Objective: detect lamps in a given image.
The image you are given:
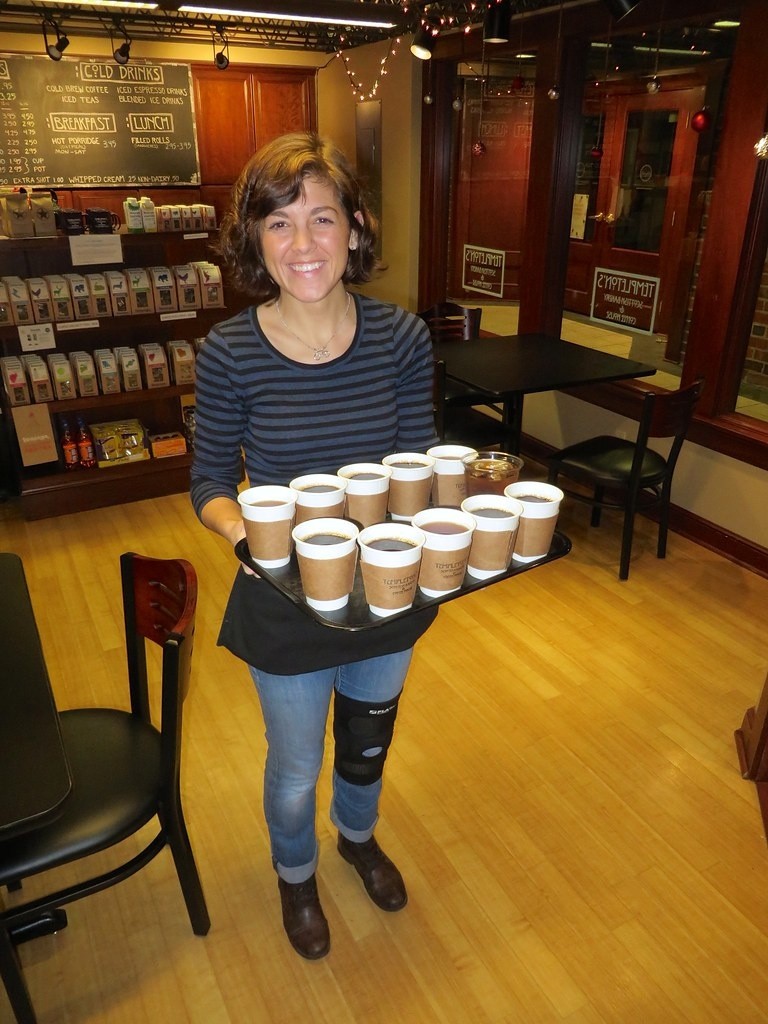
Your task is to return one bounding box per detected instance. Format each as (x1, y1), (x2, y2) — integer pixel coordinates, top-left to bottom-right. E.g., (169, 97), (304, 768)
(107, 15), (132, 65)
(482, 0), (512, 43)
(608, 0), (639, 20)
(410, 0), (444, 61)
(41, 11), (68, 60)
(213, 21), (232, 70)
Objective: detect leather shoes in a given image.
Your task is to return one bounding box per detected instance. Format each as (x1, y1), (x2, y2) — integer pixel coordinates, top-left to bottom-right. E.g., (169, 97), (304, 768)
(277, 872), (332, 961)
(337, 829), (409, 913)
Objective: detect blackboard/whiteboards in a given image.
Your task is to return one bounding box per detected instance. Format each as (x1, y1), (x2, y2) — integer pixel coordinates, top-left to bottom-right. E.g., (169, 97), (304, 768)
(0, 49), (203, 191)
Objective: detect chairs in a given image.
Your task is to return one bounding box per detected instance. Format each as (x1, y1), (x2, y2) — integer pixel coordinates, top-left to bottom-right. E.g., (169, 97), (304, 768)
(0, 550), (215, 1001)
(546, 381), (702, 582)
(414, 302), (523, 460)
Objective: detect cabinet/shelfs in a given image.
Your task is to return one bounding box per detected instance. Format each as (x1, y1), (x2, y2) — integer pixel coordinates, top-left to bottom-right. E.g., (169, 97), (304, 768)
(559, 74), (707, 333)
(55, 60), (319, 235)
(0, 223), (279, 523)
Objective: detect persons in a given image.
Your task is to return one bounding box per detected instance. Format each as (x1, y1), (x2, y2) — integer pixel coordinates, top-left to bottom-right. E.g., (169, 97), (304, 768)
(190, 131), (441, 961)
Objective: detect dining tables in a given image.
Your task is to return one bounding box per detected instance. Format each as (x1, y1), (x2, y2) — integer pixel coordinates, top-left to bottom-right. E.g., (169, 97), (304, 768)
(431, 332), (657, 458)
(0, 553), (68, 950)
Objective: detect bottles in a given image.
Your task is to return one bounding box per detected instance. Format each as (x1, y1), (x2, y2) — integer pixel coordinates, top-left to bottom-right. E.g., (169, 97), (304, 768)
(123, 196), (158, 234)
(58, 415), (97, 470)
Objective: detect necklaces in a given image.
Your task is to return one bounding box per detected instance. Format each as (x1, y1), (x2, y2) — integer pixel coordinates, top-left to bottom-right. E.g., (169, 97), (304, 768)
(273, 292), (351, 360)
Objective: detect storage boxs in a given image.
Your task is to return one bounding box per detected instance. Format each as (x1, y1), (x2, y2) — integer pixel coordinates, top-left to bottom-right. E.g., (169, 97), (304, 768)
(90, 417), (188, 469)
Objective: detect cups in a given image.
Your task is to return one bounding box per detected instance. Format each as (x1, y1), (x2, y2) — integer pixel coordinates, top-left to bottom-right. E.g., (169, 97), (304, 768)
(503, 481), (564, 564)
(336, 462), (393, 532)
(382, 452), (436, 524)
(409, 507), (478, 599)
(461, 494), (525, 579)
(237, 485), (299, 569)
(461, 451), (525, 498)
(427, 444), (479, 509)
(293, 517), (361, 612)
(358, 522), (427, 619)
(289, 474), (348, 527)
(54, 206), (122, 236)
